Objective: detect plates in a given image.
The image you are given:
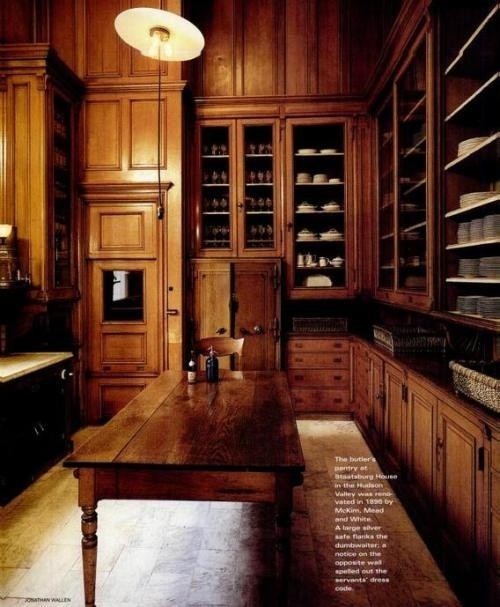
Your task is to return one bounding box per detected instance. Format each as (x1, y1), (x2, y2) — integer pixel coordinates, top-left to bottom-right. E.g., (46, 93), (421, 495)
(449, 129), (500, 321)
(291, 146), (345, 241)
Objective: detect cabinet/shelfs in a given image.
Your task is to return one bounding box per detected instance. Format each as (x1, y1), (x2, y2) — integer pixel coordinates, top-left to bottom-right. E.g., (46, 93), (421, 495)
(354, 1), (500, 606)
(74, 0), (188, 185)
(192, 95), (356, 412)
(0, 40), (84, 350)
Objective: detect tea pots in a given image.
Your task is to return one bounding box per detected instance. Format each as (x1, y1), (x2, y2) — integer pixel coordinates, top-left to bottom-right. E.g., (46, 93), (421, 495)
(297, 251), (345, 268)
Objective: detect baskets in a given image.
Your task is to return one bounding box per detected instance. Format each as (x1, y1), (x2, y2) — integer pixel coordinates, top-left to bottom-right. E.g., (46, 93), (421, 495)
(448, 359), (500, 415)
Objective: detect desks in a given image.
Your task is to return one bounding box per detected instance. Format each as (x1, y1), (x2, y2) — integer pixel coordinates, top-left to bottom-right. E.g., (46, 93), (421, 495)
(62, 369), (306, 606)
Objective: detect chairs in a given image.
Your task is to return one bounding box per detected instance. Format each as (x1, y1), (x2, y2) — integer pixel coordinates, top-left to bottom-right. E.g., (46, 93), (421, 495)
(188, 337), (245, 370)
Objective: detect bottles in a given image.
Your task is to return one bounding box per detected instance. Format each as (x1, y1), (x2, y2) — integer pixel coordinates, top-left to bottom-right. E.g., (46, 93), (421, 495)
(185, 349), (199, 384)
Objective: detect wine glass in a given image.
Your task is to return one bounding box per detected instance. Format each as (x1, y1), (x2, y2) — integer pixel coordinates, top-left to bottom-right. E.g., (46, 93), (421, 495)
(201, 139), (274, 249)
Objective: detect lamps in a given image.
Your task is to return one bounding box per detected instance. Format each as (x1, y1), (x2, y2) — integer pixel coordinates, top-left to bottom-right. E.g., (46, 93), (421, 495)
(112, 6), (205, 219)
